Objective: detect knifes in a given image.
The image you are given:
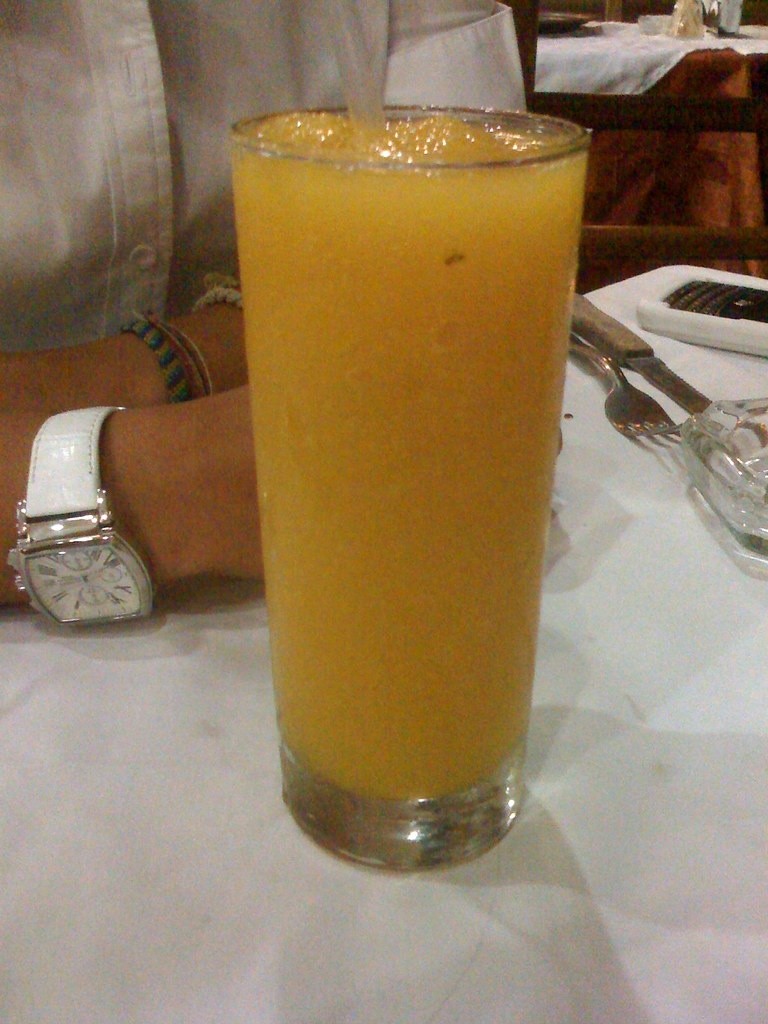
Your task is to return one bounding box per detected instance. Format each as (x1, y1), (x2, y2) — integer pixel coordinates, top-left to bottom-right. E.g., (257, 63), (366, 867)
(569, 291), (713, 417)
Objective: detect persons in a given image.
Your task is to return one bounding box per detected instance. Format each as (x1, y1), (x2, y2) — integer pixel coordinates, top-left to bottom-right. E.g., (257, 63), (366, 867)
(0, 0), (527, 608)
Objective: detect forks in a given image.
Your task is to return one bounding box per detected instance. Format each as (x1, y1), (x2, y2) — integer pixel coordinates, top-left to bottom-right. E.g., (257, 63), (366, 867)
(568, 334), (685, 437)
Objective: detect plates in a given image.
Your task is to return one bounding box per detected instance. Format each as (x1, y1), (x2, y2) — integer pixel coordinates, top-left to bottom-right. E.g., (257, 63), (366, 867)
(539, 11), (599, 33)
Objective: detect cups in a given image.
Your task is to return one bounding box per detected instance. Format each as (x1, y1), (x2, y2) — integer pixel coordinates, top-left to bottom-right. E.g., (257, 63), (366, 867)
(226, 102), (592, 869)
(718, 0), (743, 35)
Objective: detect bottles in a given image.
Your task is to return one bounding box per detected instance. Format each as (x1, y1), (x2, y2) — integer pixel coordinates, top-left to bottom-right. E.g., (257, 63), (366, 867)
(666, 0), (705, 39)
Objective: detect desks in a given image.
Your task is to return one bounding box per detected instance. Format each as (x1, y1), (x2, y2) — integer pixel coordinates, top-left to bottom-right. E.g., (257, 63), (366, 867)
(536, 25), (768, 277)
(0, 265), (767, 1024)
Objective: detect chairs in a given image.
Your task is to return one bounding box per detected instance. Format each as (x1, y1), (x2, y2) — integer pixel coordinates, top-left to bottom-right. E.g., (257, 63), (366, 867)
(504, 0), (768, 263)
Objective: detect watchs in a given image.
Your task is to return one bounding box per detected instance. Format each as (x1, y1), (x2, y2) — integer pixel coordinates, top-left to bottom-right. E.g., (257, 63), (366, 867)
(6, 407), (153, 625)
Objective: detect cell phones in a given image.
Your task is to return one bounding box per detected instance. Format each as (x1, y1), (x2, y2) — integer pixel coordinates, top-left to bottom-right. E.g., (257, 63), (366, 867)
(638, 276), (768, 360)
